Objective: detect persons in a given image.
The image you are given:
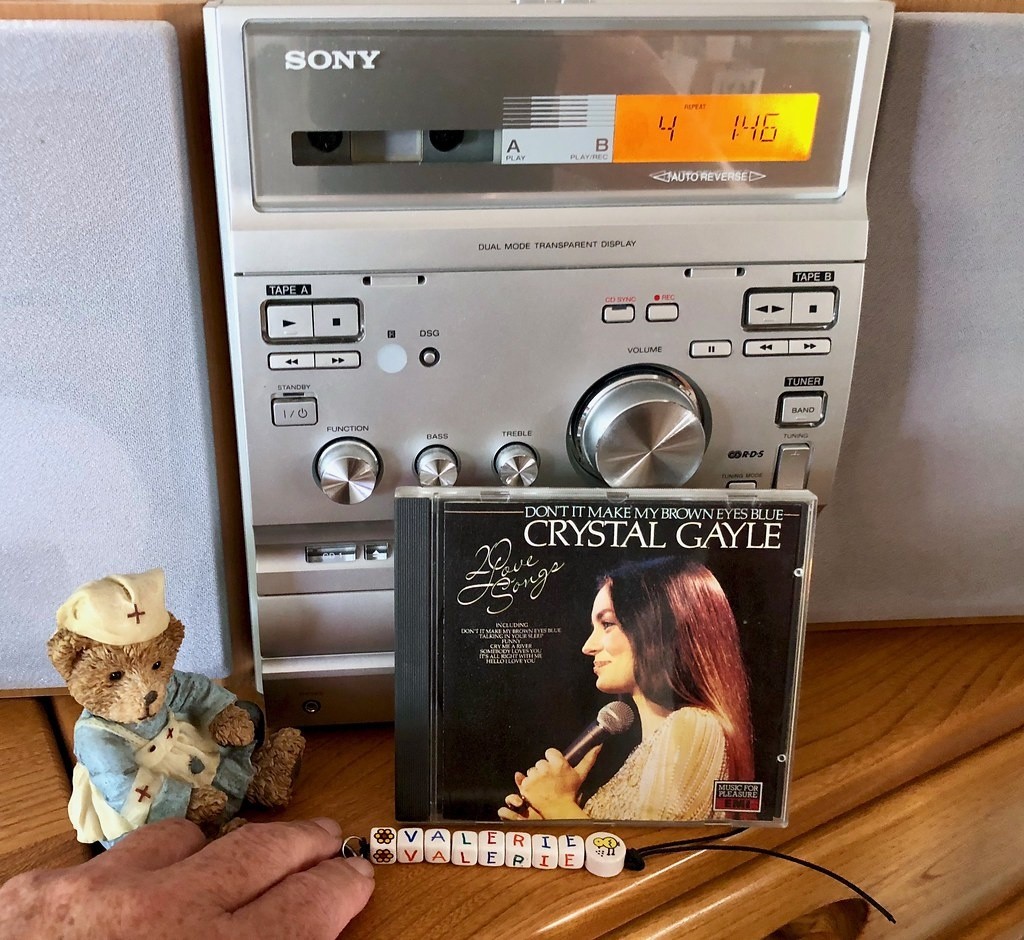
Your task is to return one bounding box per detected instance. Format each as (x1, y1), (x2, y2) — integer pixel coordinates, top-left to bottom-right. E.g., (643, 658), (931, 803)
(496, 552), (759, 822)
(0, 816), (378, 940)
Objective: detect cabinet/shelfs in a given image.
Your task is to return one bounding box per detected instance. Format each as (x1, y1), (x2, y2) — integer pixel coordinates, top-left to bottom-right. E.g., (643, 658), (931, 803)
(2, 616), (1023, 940)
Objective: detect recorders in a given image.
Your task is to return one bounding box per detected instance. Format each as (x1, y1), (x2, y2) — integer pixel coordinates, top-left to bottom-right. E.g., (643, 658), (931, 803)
(207, 0), (897, 732)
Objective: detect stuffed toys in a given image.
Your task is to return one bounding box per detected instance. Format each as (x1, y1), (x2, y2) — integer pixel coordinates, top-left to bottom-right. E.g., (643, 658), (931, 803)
(46, 569), (306, 854)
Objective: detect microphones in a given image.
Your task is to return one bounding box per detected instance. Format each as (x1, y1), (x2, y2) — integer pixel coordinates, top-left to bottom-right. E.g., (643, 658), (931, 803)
(507, 701), (633, 814)
